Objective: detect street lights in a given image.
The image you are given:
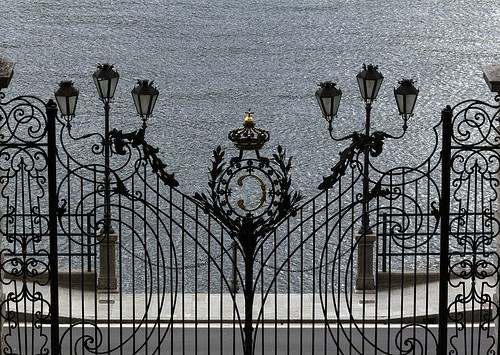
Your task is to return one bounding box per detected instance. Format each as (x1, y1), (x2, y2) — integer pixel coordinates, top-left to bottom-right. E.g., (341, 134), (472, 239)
(55, 62), (161, 295)
(313, 62), (419, 294)
